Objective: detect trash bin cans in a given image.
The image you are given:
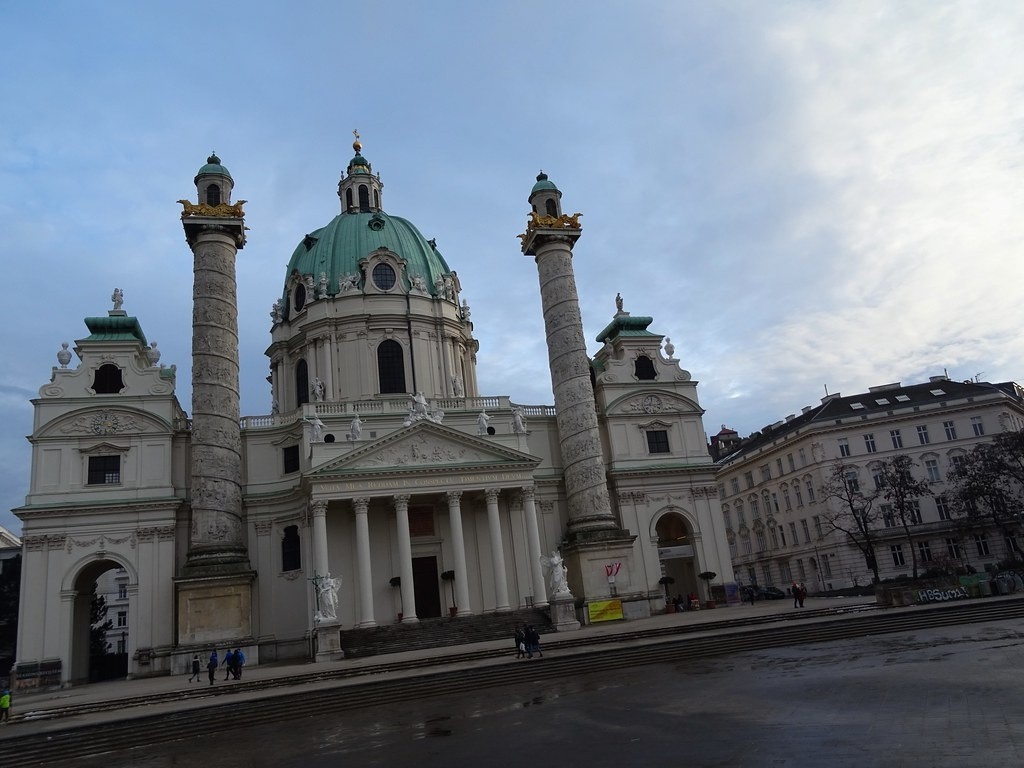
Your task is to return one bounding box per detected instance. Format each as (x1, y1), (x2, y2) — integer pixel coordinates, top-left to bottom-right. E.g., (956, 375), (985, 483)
(889, 587), (914, 606)
(978, 579), (992, 596)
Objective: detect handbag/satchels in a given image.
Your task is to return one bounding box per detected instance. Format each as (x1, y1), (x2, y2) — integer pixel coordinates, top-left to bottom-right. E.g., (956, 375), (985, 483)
(520, 641), (525, 651)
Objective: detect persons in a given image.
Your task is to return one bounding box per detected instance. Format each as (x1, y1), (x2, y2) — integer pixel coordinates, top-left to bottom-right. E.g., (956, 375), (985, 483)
(114, 288), (123, 309)
(189, 648), (246, 685)
(672, 581), (807, 608)
(515, 624), (543, 659)
(616, 293), (623, 311)
(550, 551), (564, 594)
(318, 573), (336, 618)
(271, 272), (527, 442)
(0, 691), (13, 722)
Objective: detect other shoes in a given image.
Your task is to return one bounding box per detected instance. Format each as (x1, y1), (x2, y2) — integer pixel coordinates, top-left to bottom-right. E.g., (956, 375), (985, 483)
(515, 657), (519, 659)
(527, 656), (534, 659)
(539, 654), (543, 657)
(521, 657), (525, 658)
